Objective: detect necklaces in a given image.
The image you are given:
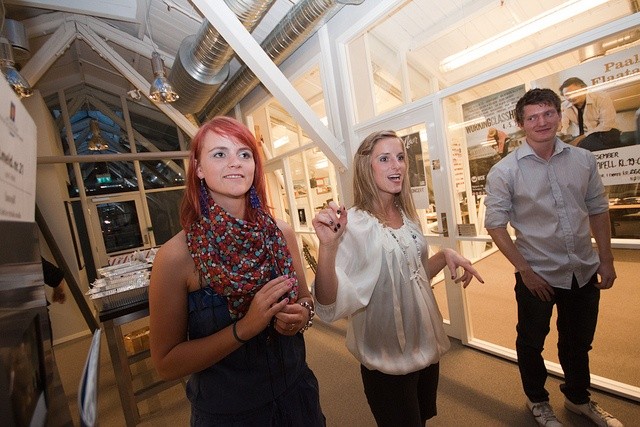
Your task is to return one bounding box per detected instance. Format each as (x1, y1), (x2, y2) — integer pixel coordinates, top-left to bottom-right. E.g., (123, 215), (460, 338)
(368, 200), (422, 273)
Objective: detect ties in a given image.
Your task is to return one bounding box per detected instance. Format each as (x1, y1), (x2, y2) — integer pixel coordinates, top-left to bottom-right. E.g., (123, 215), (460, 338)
(574, 102), (586, 136)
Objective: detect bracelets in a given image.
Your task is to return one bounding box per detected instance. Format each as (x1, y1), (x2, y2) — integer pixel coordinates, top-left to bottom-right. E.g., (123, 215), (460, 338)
(233, 321), (250, 343)
(300, 301), (315, 333)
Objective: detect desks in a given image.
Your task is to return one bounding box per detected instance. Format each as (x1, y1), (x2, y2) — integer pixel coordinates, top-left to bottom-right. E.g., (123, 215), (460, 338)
(99, 298), (184, 427)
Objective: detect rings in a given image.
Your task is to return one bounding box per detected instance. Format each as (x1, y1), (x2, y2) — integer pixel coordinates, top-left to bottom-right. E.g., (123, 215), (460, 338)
(289, 323), (295, 331)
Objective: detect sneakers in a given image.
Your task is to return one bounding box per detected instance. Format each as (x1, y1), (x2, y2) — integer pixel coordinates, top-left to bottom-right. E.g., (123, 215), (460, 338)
(525, 396), (565, 427)
(564, 397), (625, 427)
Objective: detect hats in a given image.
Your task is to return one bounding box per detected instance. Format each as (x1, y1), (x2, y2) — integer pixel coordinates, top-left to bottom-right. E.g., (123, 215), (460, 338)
(489, 127), (496, 137)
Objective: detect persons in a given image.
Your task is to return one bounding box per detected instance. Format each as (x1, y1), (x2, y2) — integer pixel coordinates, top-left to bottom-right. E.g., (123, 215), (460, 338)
(557, 77), (622, 151)
(484, 88), (623, 427)
(311, 130), (484, 427)
(487, 127), (511, 159)
(149, 117), (326, 427)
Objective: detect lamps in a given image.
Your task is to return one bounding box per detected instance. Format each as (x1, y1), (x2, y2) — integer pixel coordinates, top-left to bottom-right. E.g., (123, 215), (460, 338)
(87, 98), (110, 150)
(144, 0), (180, 103)
(0, 0), (34, 97)
